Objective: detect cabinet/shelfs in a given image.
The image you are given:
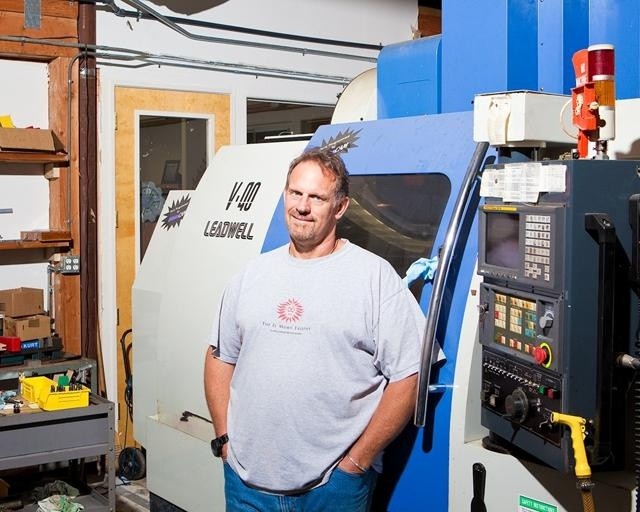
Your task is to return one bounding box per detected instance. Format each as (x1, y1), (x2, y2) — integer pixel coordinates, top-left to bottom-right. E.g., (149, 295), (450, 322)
(2, 150), (80, 372)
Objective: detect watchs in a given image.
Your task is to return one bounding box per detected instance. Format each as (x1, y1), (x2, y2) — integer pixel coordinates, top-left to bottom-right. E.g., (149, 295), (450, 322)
(201, 146), (445, 512)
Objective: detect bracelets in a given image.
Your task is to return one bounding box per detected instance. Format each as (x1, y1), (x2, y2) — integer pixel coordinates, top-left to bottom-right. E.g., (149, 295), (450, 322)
(209, 434), (230, 459)
(347, 454), (368, 476)
